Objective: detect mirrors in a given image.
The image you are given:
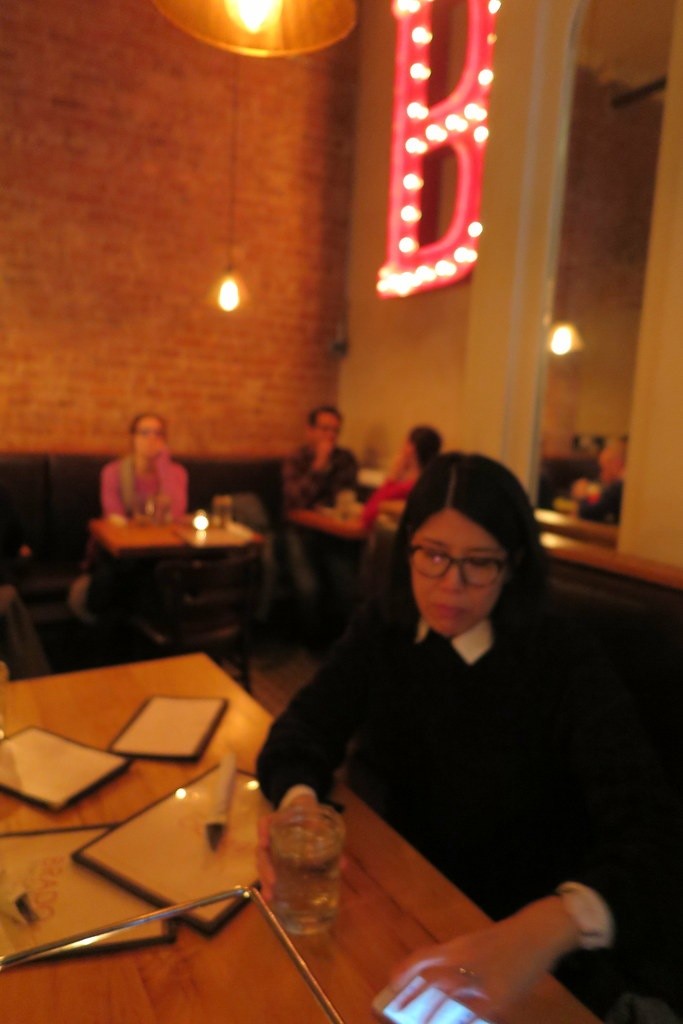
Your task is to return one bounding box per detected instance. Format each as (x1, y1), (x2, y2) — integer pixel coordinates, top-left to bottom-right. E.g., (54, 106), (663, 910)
(527, 0), (675, 556)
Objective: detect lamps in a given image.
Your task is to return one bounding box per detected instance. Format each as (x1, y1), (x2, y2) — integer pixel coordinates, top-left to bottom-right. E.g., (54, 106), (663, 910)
(219, 53), (244, 312)
(151, 0), (359, 58)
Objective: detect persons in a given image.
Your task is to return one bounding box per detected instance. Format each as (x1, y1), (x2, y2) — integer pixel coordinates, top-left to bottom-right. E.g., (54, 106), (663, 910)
(253, 453), (653, 1024)
(359, 428), (443, 526)
(280, 406), (358, 630)
(554, 435), (627, 522)
(67, 410), (189, 645)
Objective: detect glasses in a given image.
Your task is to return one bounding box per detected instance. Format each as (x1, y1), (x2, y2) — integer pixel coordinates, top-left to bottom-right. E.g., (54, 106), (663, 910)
(406, 543), (508, 589)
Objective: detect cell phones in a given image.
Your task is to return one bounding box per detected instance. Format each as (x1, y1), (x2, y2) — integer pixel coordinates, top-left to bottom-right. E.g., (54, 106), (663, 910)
(370, 971), (491, 1024)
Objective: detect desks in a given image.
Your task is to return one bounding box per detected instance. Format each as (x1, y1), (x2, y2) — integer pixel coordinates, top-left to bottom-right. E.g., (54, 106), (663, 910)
(88, 512), (266, 695)
(0, 651), (606, 1024)
(285, 504), (376, 646)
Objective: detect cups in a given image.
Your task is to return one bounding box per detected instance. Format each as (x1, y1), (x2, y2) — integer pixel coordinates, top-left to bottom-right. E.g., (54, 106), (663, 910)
(212, 495), (233, 528)
(262, 803), (346, 936)
(333, 489), (355, 522)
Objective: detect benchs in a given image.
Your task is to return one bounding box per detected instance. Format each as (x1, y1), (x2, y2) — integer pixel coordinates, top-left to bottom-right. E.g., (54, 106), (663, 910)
(0, 451), (289, 637)
(356, 522), (683, 1024)
